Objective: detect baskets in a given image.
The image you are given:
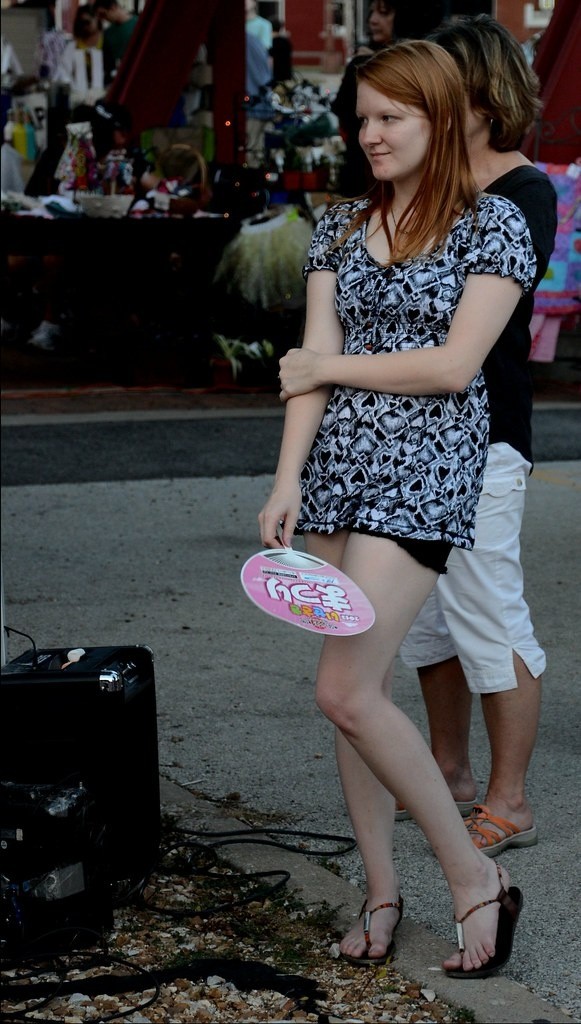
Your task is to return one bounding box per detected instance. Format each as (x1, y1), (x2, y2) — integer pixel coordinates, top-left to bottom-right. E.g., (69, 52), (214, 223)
(139, 139), (214, 215)
(78, 169), (135, 221)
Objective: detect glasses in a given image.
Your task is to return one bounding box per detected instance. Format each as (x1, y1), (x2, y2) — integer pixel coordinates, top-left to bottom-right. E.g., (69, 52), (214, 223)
(96, 9), (110, 21)
(83, 19), (91, 25)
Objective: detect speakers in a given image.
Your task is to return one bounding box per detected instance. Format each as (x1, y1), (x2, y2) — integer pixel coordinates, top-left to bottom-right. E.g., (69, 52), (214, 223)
(0, 646), (163, 884)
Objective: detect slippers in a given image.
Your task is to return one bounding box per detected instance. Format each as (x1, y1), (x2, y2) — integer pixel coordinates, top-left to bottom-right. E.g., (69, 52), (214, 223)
(434, 804), (539, 857)
(394, 796), (478, 821)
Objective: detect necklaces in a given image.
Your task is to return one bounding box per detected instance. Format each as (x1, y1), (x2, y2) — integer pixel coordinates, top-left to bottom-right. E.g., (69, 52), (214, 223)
(391, 208), (409, 234)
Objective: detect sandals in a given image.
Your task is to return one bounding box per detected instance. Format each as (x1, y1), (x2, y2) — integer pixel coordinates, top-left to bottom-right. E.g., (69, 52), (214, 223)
(441, 858), (525, 979)
(339, 892), (405, 967)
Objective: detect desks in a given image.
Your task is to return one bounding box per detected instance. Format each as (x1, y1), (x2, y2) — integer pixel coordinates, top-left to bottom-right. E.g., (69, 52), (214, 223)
(0, 197), (229, 388)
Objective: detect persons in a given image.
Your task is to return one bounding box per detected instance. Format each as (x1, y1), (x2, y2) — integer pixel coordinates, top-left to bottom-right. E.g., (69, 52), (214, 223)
(328, 0), (429, 198)
(395, 12), (560, 858)
(54, 1), (140, 105)
(245, 0), (294, 95)
(256, 40), (525, 978)
